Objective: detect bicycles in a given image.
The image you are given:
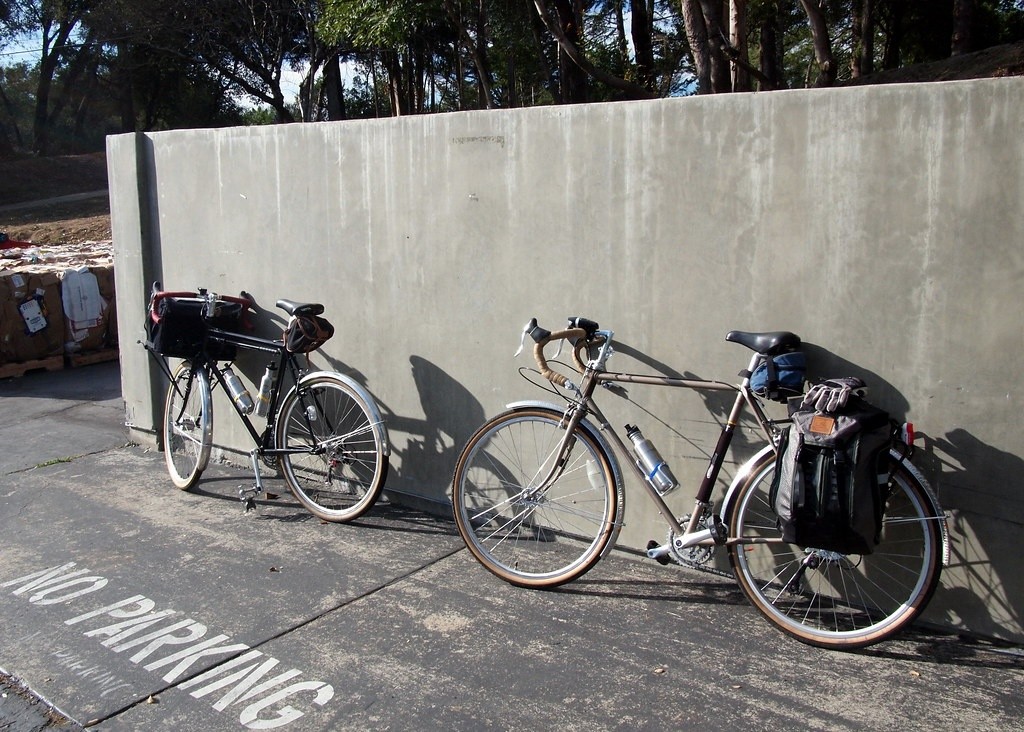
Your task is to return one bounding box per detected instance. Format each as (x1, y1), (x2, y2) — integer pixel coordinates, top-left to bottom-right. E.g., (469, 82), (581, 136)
(451, 317), (952, 652)
(144, 280), (392, 524)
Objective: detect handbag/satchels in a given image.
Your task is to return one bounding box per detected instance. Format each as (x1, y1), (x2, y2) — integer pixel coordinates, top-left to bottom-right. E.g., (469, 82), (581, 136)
(282, 316), (335, 369)
(737, 351), (809, 404)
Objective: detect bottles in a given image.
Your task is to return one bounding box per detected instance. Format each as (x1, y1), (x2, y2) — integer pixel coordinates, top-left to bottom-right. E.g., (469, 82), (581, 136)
(623, 423), (677, 493)
(254, 361), (279, 417)
(222, 363), (253, 414)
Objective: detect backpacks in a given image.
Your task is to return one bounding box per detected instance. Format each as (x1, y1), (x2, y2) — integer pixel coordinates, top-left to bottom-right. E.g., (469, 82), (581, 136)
(769, 411), (885, 556)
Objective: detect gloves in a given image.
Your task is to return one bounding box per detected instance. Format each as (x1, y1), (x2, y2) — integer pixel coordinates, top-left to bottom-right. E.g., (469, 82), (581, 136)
(799, 375), (869, 414)
